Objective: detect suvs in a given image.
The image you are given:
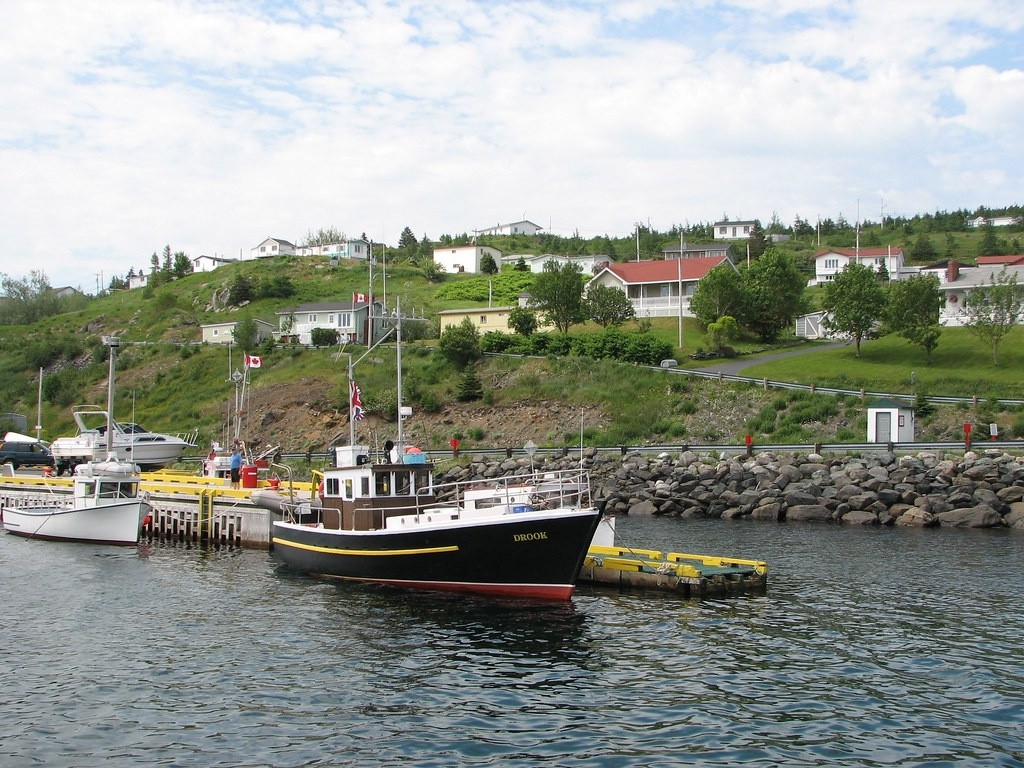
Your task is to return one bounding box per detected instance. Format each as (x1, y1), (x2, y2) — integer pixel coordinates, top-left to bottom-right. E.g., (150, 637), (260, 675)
(0, 440), (54, 470)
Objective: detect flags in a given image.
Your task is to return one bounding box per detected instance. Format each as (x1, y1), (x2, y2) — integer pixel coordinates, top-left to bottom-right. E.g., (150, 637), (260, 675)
(351, 380), (365, 421)
(245, 354), (263, 369)
(354, 293), (368, 303)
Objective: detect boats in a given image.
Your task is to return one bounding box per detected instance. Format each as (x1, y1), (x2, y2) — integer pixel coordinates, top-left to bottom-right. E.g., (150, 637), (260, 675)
(46, 405), (200, 473)
(1, 388), (154, 547)
(270, 295), (609, 600)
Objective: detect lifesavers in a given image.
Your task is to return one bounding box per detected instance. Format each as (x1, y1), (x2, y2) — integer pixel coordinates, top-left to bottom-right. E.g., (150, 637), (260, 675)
(319, 481), (324, 500)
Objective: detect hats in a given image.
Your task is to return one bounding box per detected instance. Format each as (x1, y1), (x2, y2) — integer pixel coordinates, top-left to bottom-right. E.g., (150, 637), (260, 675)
(231, 446), (236, 450)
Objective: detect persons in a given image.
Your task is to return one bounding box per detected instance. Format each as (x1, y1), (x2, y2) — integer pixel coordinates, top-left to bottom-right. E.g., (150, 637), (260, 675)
(229, 447), (243, 490)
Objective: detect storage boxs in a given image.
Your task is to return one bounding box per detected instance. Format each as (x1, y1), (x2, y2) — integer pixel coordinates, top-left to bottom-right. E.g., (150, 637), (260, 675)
(403, 452), (427, 464)
(513, 505), (533, 513)
